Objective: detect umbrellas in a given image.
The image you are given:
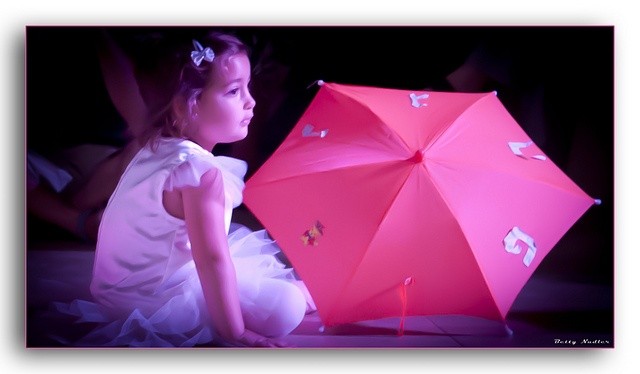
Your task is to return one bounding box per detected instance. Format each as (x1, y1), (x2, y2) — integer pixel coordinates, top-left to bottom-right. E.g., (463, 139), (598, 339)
(242, 79), (601, 336)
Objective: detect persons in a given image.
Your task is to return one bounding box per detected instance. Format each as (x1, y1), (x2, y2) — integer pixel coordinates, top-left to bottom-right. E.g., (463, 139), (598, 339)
(46, 31), (318, 346)
(26, 27), (152, 245)
